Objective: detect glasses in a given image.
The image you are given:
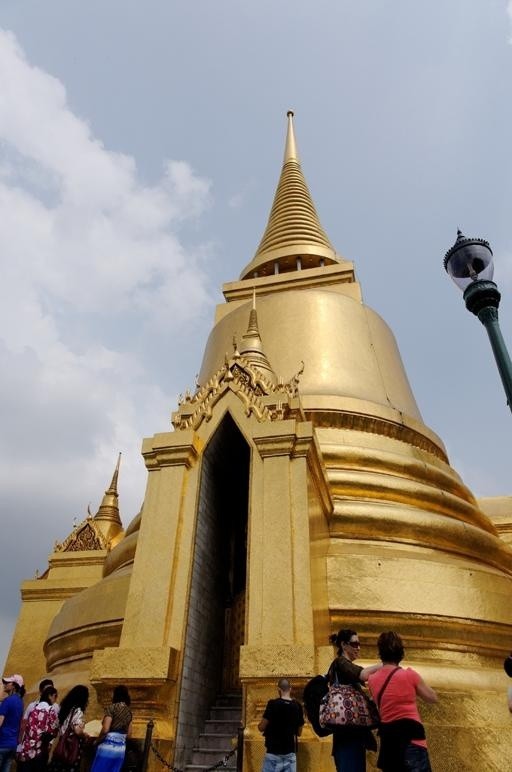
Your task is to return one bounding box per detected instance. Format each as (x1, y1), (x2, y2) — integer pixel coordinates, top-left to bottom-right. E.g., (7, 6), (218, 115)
(346, 641), (359, 648)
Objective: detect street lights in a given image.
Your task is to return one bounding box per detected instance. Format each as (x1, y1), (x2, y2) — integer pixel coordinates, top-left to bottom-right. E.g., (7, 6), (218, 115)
(444, 226), (511, 410)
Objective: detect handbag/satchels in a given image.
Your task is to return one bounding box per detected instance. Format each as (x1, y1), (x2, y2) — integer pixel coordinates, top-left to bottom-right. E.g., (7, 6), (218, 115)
(318, 682), (379, 730)
(46, 727), (81, 767)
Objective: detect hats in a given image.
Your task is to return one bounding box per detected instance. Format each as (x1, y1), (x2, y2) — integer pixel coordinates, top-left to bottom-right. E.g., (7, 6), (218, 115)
(2, 674), (25, 688)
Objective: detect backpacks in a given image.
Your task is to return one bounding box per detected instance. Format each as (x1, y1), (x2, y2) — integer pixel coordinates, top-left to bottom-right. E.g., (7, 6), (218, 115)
(302, 674), (335, 737)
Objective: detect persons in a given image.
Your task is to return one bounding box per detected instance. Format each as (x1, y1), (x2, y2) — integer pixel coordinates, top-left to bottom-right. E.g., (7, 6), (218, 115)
(326, 629), (384, 772)
(0, 673), (133, 771)
(258, 678), (304, 772)
(367, 629), (439, 771)
